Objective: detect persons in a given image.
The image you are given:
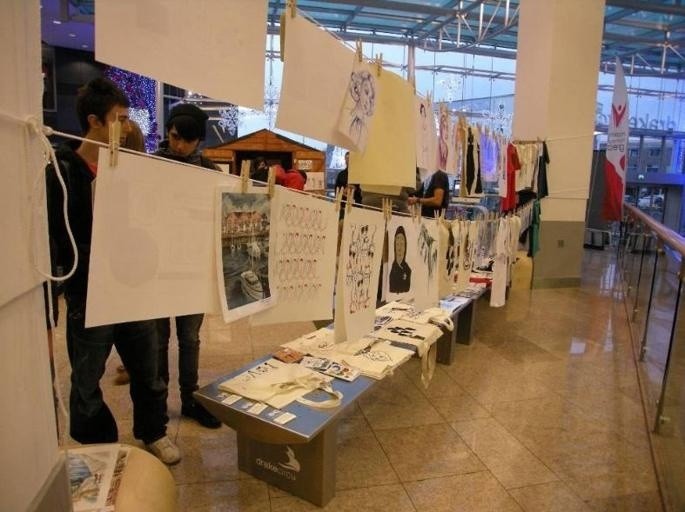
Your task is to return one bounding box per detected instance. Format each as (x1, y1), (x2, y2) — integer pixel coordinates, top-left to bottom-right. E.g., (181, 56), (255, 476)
(57, 76), (180, 464)
(250, 151), (449, 218)
(278, 443), (302, 473)
(389, 226), (411, 293)
(345, 70), (375, 146)
(417, 104), (429, 148)
(348, 226), (375, 312)
(139, 105), (223, 429)
(95, 119), (148, 385)
(438, 115), (448, 171)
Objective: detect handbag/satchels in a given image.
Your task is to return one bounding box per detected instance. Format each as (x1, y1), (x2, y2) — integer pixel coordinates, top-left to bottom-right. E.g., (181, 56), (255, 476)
(218, 358), (343, 410)
(410, 308), (455, 332)
(375, 319), (444, 357)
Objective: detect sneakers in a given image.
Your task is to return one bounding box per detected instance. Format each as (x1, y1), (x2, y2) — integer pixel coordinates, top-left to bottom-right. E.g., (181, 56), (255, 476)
(181, 400), (221, 428)
(147, 436), (180, 464)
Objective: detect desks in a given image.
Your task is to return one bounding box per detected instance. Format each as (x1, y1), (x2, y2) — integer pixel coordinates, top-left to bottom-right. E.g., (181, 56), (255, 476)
(192, 295), (461, 510)
(445, 252), (515, 351)
